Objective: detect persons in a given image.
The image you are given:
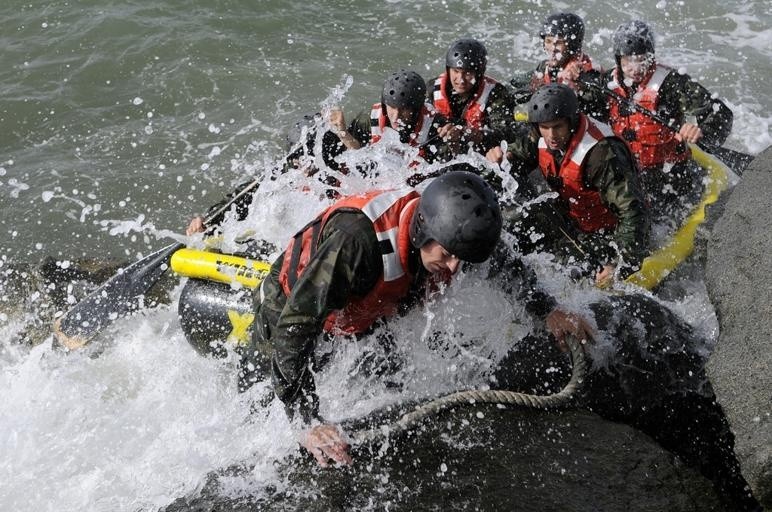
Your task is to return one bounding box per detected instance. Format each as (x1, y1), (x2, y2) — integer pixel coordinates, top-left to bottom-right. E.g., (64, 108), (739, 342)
(347, 68), (461, 184)
(185, 107), (368, 253)
(422, 39), (516, 178)
(487, 82), (645, 295)
(501, 12), (603, 103)
(232, 172), (587, 471)
(563, 21), (733, 230)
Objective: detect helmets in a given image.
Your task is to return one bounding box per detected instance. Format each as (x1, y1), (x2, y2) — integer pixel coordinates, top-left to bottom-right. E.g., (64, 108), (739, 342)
(380, 69), (427, 127)
(407, 168), (502, 266)
(611, 19), (656, 66)
(539, 12), (585, 56)
(524, 85), (579, 138)
(444, 37), (488, 83)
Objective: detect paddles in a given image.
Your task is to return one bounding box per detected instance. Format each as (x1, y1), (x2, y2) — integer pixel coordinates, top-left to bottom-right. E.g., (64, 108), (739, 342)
(51, 137), (311, 351)
(576, 75), (754, 177)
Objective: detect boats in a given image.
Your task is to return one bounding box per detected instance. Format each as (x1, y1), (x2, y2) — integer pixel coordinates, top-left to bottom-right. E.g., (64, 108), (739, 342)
(179, 114), (756, 369)
